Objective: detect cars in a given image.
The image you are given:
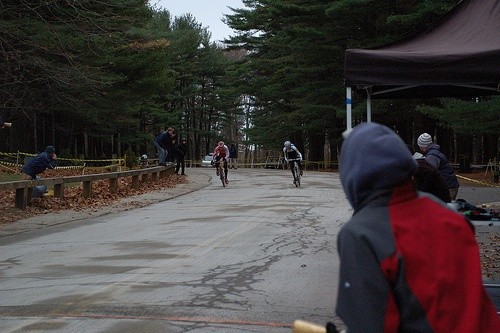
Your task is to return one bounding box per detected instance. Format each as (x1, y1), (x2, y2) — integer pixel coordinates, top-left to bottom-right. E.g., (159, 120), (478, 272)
(201, 155), (214, 168)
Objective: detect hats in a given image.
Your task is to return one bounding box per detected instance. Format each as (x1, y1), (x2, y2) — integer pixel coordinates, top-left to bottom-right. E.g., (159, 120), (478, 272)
(417, 133), (433, 147)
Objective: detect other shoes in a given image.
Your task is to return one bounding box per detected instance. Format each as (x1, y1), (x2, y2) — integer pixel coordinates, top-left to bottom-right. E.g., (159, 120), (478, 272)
(293, 178), (296, 184)
(300, 170), (303, 176)
(181, 173), (186, 176)
(216, 169), (219, 176)
(225, 179), (229, 184)
(175, 172), (180, 175)
(158, 163), (167, 166)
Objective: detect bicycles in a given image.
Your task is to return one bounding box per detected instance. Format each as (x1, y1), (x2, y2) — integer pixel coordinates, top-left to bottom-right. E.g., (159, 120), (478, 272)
(211, 158), (229, 187)
(286, 158), (302, 187)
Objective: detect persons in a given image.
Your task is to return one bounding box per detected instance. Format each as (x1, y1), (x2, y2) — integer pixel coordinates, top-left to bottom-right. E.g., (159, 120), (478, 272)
(335, 123), (500, 333)
(283, 141), (303, 183)
(19, 146), (57, 206)
(410, 133), (459, 205)
(154, 127), (187, 176)
(211, 141), (230, 184)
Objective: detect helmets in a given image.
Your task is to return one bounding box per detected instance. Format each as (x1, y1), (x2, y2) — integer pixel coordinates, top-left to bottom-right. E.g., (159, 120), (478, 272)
(218, 141), (224, 147)
(284, 141), (291, 146)
